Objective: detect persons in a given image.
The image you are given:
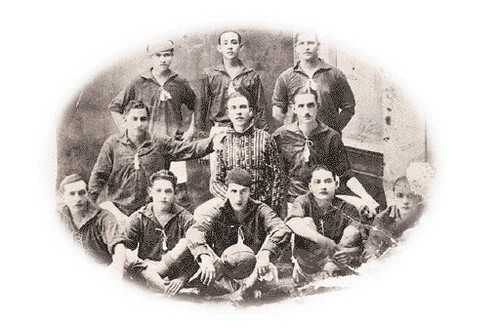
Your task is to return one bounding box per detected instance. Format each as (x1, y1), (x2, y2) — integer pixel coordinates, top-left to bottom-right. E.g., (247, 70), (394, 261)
(195, 30), (273, 142)
(107, 40), (197, 142)
(361, 175), (425, 263)
(272, 85), (383, 215)
(192, 88), (281, 218)
(186, 168), (290, 307)
(283, 158), (367, 288)
(56, 173), (128, 280)
(88, 102), (227, 217)
(284, 164), (369, 298)
(272, 33), (356, 138)
(124, 170), (200, 298)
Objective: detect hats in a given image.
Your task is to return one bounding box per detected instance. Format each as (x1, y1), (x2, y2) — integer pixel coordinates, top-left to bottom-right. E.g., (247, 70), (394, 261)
(147, 40), (174, 58)
(224, 167), (252, 188)
(62, 173), (82, 185)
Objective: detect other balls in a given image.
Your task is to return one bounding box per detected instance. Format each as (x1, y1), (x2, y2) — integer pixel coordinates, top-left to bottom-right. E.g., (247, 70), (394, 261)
(221, 243), (257, 279)
